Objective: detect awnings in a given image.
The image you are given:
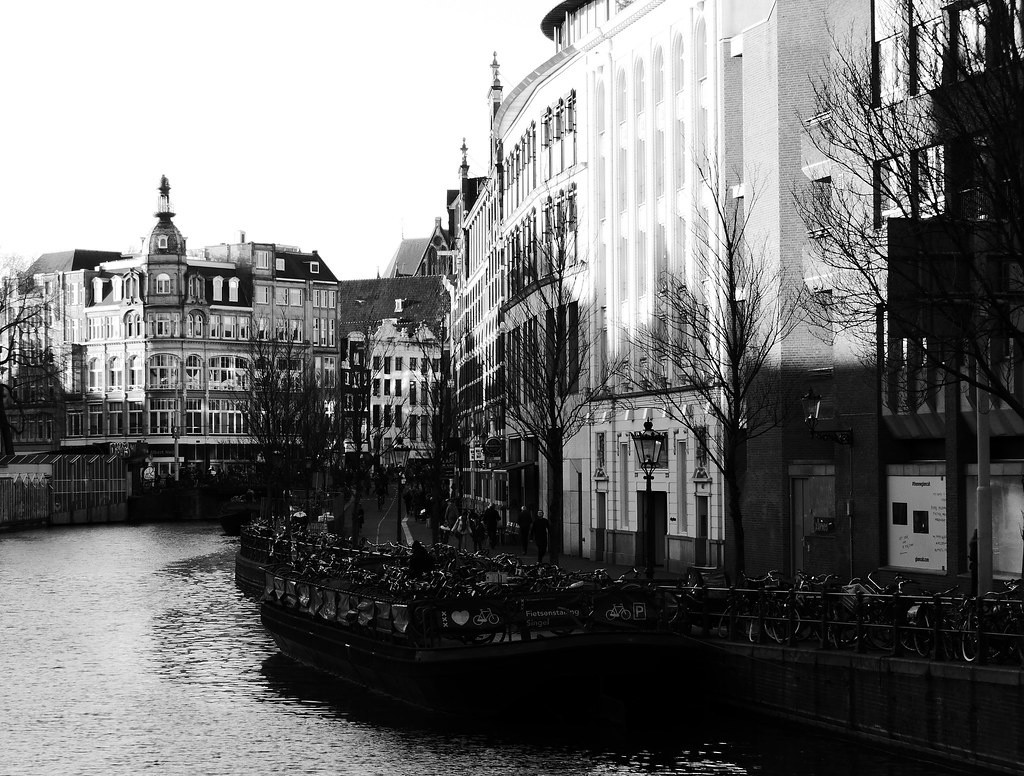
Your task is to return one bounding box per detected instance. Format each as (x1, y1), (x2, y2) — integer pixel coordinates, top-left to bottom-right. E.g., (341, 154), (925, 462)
(478, 461), (535, 473)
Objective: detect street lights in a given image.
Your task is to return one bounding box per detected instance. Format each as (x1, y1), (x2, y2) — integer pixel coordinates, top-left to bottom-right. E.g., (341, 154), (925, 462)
(389, 436), (411, 541)
(628, 419), (669, 577)
(304, 455), (315, 524)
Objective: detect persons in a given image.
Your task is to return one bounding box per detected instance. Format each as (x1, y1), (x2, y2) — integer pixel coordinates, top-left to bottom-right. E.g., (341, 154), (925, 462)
(380, 463), (502, 549)
(291, 507), (309, 530)
(518, 505), (532, 556)
(529, 510), (552, 564)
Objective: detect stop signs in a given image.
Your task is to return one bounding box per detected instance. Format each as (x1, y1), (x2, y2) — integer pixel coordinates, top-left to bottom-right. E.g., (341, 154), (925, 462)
(487, 438), (501, 454)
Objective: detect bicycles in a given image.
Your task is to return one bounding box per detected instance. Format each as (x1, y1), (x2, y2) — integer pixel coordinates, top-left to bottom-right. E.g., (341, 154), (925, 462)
(242, 490), (1023, 666)
